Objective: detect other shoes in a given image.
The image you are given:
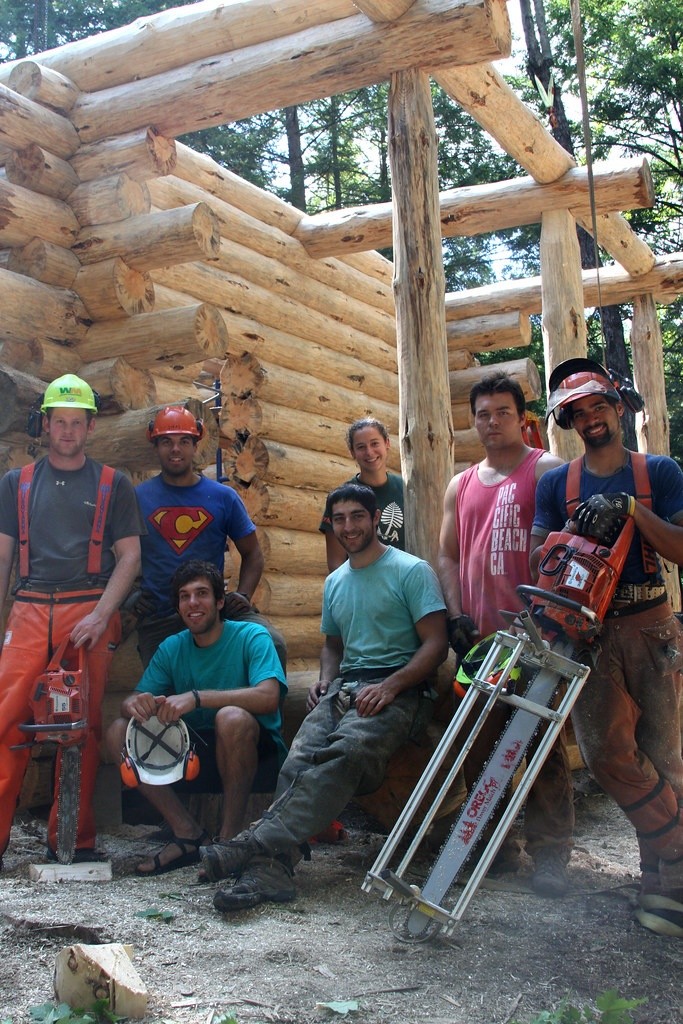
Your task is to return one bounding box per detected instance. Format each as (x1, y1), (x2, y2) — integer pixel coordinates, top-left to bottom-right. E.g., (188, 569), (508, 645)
(645, 891), (682, 936)
(425, 807), (461, 859)
(46, 847), (106, 862)
(533, 847), (569, 894)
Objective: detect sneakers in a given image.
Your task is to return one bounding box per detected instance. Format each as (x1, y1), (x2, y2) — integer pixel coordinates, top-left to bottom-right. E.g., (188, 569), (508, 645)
(198, 829), (261, 882)
(212, 851), (296, 911)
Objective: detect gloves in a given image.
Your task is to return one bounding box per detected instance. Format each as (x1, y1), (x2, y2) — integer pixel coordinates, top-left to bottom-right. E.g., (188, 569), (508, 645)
(120, 584), (159, 622)
(222, 591), (251, 620)
(447, 613), (480, 657)
(569, 491), (636, 538)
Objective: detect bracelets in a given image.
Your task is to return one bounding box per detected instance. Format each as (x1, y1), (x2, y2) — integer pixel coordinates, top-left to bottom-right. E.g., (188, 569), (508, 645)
(192, 689), (201, 709)
(565, 519), (573, 534)
(239, 592), (251, 603)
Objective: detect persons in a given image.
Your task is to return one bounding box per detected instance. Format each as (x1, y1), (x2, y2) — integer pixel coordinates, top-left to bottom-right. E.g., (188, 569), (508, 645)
(198, 485), (448, 912)
(319, 369), (576, 898)
(530, 358), (683, 924)
(0, 374), (288, 876)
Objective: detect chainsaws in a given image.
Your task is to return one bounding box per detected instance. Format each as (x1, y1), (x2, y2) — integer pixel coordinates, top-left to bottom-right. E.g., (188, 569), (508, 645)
(359, 505), (636, 945)
(8, 632), (89, 865)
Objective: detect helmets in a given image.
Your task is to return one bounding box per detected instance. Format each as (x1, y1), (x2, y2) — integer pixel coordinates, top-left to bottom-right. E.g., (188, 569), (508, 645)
(145, 405), (207, 441)
(39, 374), (98, 417)
(119, 709), (201, 788)
(551, 370), (620, 409)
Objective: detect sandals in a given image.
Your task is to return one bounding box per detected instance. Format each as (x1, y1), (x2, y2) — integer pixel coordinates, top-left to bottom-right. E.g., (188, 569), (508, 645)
(134, 829), (210, 876)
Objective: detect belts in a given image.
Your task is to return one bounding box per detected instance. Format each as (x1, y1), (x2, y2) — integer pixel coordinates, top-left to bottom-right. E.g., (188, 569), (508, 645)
(604, 590), (668, 620)
(336, 668), (396, 682)
